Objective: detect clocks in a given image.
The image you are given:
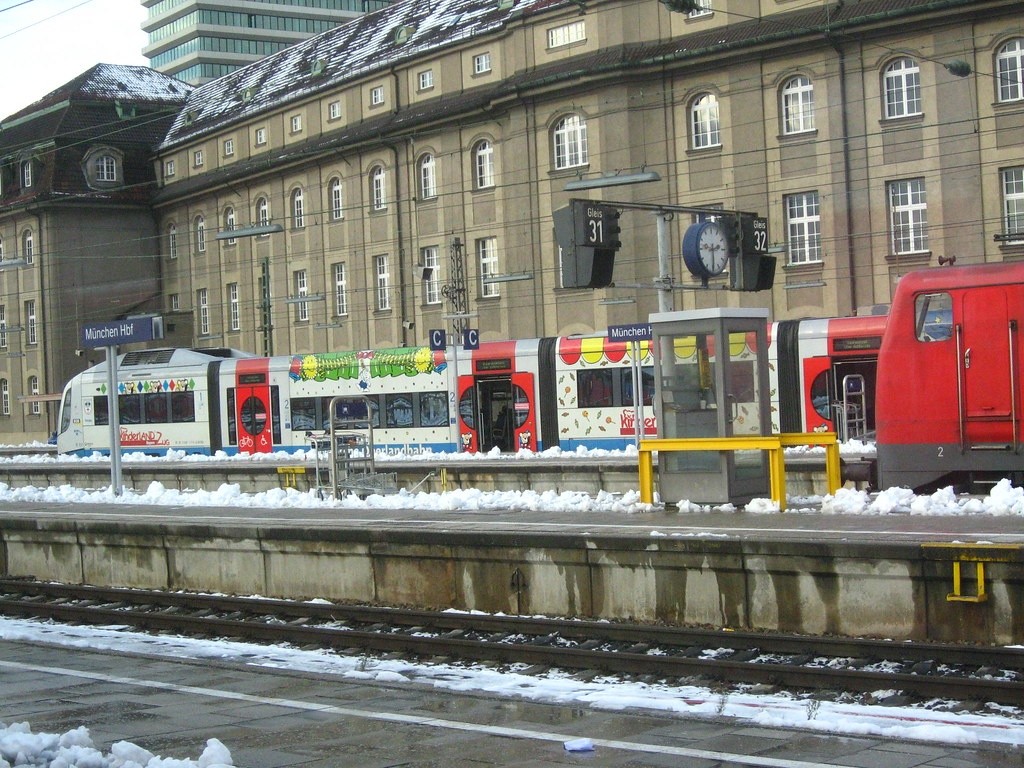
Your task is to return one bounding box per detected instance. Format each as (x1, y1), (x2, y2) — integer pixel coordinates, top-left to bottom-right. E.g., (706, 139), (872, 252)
(683, 214), (730, 285)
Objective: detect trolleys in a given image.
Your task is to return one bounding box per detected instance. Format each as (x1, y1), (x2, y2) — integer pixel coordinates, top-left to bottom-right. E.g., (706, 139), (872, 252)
(301, 392), (399, 502)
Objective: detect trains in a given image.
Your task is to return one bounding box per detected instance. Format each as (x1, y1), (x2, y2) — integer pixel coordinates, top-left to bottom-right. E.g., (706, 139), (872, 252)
(48, 308), (953, 456)
(840, 259), (1023, 494)
